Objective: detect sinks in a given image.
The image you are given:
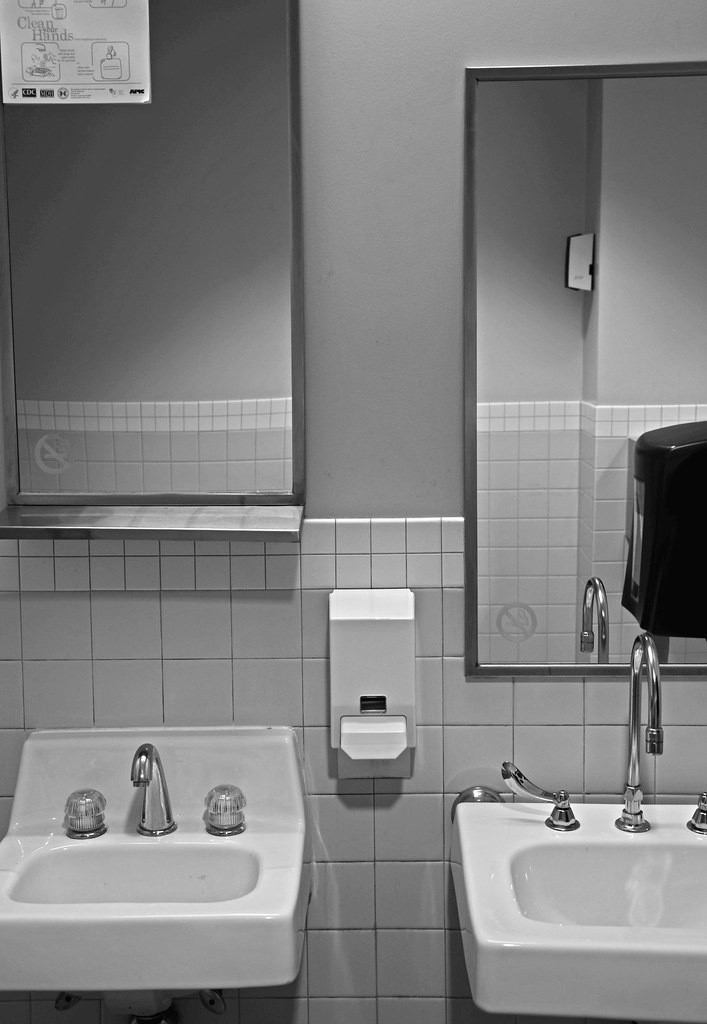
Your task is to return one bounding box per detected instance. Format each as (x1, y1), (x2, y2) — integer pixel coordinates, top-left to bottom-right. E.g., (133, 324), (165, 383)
(0, 723), (316, 992)
(451, 800), (707, 1024)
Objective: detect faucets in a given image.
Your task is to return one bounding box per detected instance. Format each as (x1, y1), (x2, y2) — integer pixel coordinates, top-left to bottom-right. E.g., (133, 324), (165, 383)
(613, 632), (667, 834)
(130, 743), (179, 838)
(577, 576), (611, 665)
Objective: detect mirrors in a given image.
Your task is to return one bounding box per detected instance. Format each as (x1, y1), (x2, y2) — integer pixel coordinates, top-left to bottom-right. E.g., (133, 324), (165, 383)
(462, 60), (707, 680)
(0, 0), (306, 507)
